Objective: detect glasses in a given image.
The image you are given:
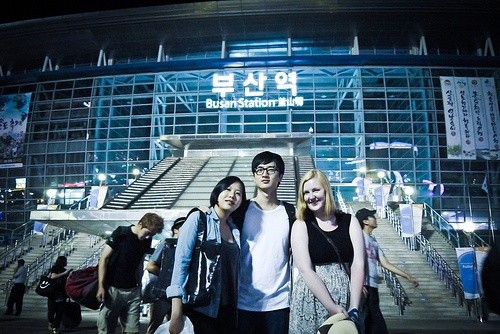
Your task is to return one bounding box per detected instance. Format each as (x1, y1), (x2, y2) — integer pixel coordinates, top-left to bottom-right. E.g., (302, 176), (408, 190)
(254, 167), (279, 175)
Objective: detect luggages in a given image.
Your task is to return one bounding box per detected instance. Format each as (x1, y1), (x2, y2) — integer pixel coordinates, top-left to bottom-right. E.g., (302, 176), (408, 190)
(61, 297), (82, 329)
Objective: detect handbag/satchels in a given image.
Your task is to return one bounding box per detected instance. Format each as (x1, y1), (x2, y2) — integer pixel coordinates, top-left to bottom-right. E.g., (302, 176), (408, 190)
(66, 264), (103, 310)
(152, 207), (222, 309)
(35, 269), (57, 297)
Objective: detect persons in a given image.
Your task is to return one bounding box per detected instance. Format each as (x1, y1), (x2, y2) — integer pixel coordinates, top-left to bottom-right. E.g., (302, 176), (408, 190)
(96, 213), (164, 334)
(3, 259), (27, 316)
(198, 151), (296, 334)
(146, 217), (186, 334)
(291, 169), (365, 334)
(47, 256), (73, 334)
(355, 208), (419, 334)
(166, 176), (246, 334)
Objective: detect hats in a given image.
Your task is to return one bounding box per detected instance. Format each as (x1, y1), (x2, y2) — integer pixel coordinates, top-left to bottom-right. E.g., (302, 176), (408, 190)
(355, 208), (377, 220)
(317, 313), (358, 334)
(171, 217), (186, 231)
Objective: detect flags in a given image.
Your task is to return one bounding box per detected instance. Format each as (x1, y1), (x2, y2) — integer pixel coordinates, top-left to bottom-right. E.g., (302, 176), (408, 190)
(481, 177), (488, 193)
